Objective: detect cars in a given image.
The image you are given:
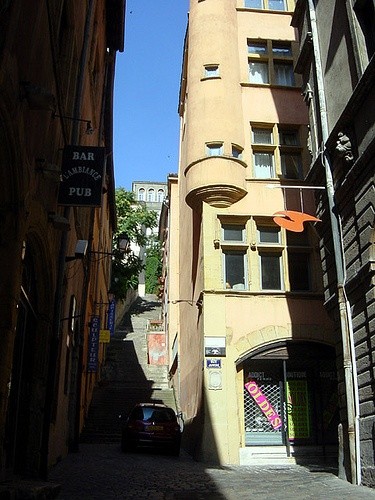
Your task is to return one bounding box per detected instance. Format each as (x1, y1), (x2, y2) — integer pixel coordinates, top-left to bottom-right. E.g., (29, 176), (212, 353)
(122, 404), (180, 459)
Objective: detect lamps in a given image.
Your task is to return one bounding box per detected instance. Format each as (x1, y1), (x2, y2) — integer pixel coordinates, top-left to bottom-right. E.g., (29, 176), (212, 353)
(93, 287), (115, 305)
(51, 111), (94, 136)
(88, 231), (132, 260)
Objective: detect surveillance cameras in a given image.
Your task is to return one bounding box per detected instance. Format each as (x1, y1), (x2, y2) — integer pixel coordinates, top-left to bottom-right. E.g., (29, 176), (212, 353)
(74, 240), (88, 258)
(164, 199), (169, 209)
